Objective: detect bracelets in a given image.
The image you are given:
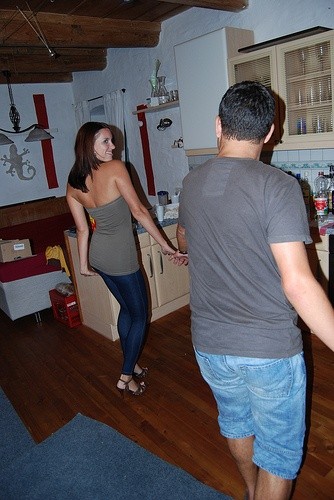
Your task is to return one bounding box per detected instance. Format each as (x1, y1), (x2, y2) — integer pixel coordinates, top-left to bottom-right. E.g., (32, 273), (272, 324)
(178, 248), (188, 254)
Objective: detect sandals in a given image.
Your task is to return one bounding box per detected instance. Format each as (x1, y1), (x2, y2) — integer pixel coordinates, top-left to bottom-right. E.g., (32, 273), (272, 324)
(116, 369), (149, 400)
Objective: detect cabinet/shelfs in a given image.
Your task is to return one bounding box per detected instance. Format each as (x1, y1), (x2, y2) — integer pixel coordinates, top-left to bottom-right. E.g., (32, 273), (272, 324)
(302, 232), (329, 297)
(67, 223), (191, 341)
(174, 26), (254, 150)
(227, 30), (334, 145)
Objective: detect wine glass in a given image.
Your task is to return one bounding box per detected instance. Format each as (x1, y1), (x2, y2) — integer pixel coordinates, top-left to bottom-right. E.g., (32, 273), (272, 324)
(298, 43), (327, 75)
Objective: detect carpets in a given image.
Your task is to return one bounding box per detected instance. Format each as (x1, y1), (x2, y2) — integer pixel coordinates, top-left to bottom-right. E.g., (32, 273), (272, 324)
(0, 387), (235, 500)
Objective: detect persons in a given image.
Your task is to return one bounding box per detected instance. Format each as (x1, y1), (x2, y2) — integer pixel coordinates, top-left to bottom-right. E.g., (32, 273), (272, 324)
(168, 80), (334, 500)
(66, 120), (175, 400)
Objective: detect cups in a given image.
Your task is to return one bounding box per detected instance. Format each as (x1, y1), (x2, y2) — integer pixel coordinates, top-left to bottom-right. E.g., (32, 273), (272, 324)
(168, 90), (178, 101)
(157, 191), (168, 205)
(297, 77), (333, 135)
(171, 195), (179, 203)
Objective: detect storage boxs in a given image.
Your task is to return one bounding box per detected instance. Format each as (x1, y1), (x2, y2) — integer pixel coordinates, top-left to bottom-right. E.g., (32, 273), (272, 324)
(0, 238), (32, 263)
(49, 288), (81, 328)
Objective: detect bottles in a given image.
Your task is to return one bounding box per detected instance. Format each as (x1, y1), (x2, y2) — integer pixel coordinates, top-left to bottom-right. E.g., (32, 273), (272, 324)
(313, 172), (334, 221)
(147, 76), (170, 105)
(156, 204), (164, 221)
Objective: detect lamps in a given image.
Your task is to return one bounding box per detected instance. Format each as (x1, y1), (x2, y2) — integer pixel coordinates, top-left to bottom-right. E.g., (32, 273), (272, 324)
(0, 70), (55, 145)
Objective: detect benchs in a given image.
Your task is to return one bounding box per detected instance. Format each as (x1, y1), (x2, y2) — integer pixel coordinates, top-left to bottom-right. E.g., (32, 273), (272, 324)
(0, 212), (74, 324)
